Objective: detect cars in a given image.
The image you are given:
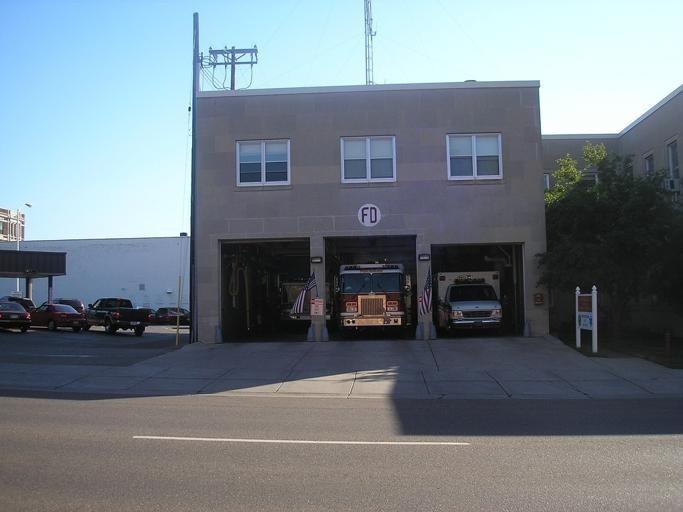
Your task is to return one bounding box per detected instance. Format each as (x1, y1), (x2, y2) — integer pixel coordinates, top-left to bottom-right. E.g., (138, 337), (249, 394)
(0, 297), (190, 336)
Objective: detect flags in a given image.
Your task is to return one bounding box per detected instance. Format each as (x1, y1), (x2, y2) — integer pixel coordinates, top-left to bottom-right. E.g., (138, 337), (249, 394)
(418, 263), (434, 317)
(289, 269), (316, 316)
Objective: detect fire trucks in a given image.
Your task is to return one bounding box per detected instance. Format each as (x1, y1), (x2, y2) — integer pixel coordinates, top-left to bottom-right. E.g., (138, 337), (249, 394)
(433, 271), (502, 329)
(334, 264), (412, 341)
